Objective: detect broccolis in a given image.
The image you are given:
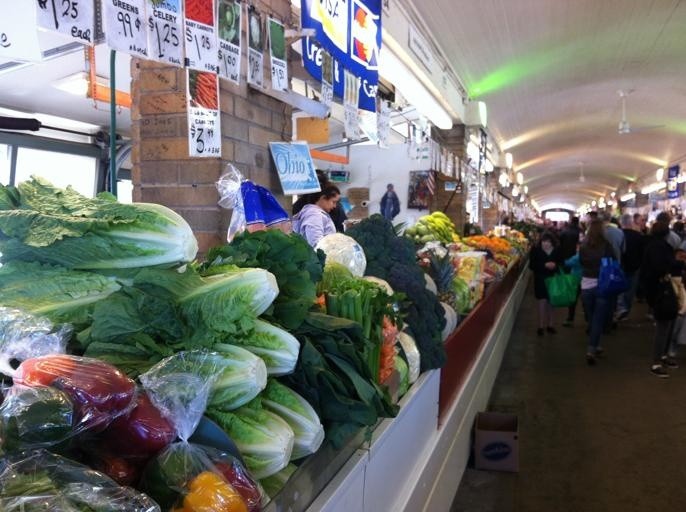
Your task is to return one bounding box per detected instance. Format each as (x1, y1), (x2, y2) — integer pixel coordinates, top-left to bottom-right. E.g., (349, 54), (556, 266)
(343, 214), (447, 372)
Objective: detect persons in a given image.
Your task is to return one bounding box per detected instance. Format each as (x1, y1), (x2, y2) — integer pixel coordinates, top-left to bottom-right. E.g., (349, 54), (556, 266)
(462, 202), (686, 380)
(380, 183), (401, 222)
(290, 186), (340, 247)
(292, 167), (347, 233)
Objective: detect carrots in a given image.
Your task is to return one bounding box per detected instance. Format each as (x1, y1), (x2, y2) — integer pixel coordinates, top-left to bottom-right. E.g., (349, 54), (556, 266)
(191, 72), (217, 110)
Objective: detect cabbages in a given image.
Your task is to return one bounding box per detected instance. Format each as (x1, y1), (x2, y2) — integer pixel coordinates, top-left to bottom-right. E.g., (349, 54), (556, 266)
(0, 173), (327, 413)
(201, 379), (324, 511)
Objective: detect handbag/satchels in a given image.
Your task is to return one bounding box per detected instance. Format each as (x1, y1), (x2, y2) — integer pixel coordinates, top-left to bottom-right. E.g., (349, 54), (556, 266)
(596, 244), (632, 298)
(543, 264), (583, 308)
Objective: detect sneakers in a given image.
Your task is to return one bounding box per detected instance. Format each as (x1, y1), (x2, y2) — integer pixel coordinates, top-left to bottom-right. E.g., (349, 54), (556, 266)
(562, 319), (576, 328)
(656, 356), (681, 369)
(668, 340), (679, 357)
(547, 326), (554, 334)
(583, 346), (607, 366)
(647, 362), (671, 379)
(612, 308), (633, 323)
(536, 327), (545, 337)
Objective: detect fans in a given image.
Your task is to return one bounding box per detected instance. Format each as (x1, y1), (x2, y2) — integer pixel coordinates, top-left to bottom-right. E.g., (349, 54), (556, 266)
(617, 88), (665, 135)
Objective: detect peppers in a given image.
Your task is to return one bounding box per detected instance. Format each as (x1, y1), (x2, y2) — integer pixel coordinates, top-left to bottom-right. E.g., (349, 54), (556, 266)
(0, 354), (261, 511)
